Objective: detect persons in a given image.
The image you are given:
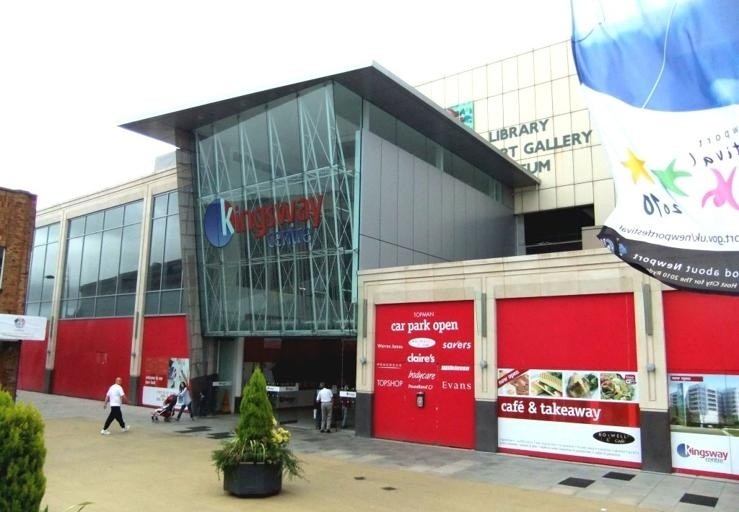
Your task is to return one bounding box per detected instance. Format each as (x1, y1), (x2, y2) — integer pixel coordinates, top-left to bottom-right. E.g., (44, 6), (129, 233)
(175, 381), (194, 420)
(314, 382), (355, 432)
(101, 377), (131, 434)
(197, 392), (208, 416)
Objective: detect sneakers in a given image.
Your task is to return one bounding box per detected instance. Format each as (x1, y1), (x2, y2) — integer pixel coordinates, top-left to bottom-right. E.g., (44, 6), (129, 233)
(100, 425), (130, 435)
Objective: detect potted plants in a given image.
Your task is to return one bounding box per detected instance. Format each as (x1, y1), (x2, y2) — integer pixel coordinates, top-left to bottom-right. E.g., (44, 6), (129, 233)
(211, 368), (311, 497)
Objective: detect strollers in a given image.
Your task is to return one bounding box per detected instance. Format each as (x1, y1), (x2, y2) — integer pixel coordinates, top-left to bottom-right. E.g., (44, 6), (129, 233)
(150, 394), (177, 424)
